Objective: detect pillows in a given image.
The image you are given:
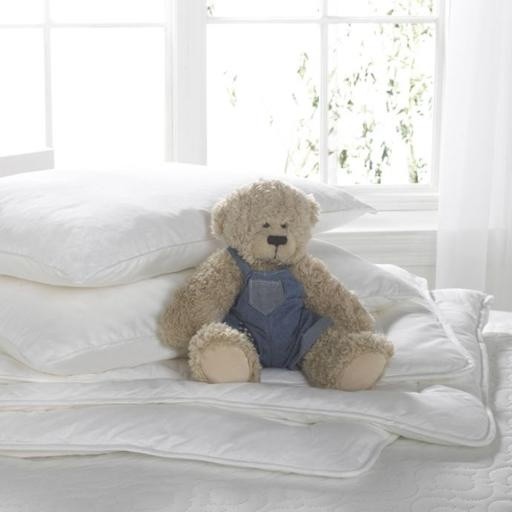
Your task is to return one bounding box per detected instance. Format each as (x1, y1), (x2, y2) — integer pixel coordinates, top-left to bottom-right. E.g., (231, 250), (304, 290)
(0, 158), (376, 289)
(0, 240), (425, 376)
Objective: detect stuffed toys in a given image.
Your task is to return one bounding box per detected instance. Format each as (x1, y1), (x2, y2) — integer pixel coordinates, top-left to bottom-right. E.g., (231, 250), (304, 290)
(156, 180), (395, 390)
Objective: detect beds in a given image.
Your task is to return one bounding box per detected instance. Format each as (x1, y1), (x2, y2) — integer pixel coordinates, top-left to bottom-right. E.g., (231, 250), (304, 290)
(0, 147), (512, 512)
